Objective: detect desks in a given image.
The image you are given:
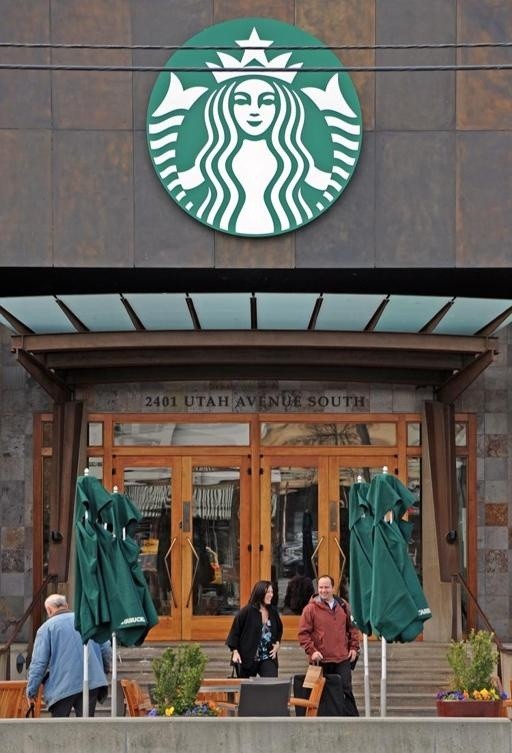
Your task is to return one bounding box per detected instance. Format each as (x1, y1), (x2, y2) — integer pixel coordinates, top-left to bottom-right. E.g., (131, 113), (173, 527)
(138, 680), (241, 717)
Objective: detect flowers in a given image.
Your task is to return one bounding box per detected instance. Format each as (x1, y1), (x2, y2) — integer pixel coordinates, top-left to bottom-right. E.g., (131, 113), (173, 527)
(437, 686), (507, 703)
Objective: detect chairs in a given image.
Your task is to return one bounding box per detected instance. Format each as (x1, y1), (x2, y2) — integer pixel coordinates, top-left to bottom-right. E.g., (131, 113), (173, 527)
(121, 677), (157, 716)
(2, 680), (44, 718)
(214, 674), (294, 716)
(289, 676), (328, 717)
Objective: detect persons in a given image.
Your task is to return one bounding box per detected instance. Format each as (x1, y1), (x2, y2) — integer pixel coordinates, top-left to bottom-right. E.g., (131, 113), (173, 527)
(298, 575), (360, 715)
(225, 582), (284, 678)
(176, 79), (331, 232)
(25, 592), (110, 718)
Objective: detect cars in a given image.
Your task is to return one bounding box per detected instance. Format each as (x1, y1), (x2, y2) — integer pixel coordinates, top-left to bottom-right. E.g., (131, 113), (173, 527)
(279, 533), (319, 581)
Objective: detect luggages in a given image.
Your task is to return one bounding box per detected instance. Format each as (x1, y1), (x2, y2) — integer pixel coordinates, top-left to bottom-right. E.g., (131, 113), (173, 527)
(292, 674), (344, 717)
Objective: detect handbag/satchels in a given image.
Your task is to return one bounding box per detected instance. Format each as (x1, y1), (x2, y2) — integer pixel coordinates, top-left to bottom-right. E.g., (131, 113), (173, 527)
(349, 647), (359, 670)
(227, 659), (241, 704)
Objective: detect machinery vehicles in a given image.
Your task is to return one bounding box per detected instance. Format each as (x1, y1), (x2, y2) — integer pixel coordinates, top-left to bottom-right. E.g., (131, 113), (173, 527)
(130, 526), (220, 593)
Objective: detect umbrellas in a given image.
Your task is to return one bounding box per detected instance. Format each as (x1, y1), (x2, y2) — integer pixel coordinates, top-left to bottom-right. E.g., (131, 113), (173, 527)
(348, 474), (379, 718)
(105, 486), (159, 718)
(75, 466), (116, 718)
(368, 465), (433, 717)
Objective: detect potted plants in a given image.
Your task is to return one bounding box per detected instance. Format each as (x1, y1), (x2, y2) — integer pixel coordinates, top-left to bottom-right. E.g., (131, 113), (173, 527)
(435, 627), (508, 717)
